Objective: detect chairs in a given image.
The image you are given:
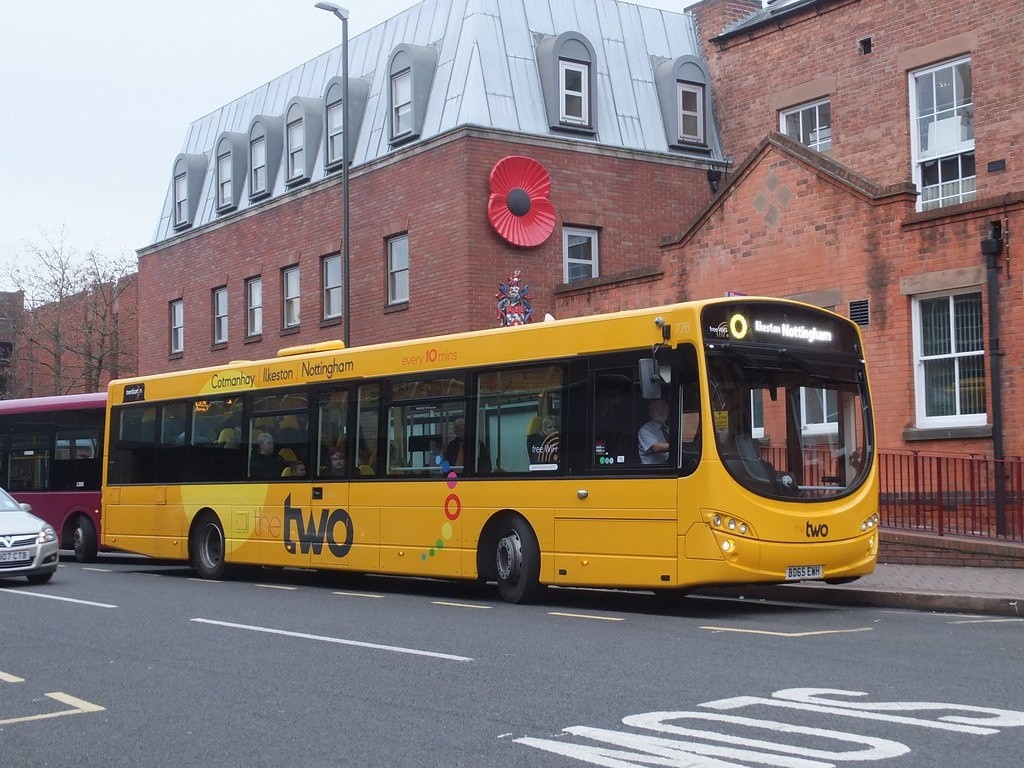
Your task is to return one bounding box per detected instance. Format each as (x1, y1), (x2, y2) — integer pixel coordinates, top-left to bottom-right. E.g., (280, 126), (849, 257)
(218, 426), (410, 478)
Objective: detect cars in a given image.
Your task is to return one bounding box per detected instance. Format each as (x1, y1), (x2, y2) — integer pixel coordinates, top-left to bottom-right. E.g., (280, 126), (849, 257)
(0, 488), (59, 583)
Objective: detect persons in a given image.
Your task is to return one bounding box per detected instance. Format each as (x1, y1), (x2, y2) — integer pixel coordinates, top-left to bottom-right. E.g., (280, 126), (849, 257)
(165, 399), (372, 478)
(494, 269), (533, 328)
(532, 415), (561, 472)
(372, 439), (406, 475)
(637, 398), (671, 465)
(445, 418), (492, 475)
(17, 466), (36, 491)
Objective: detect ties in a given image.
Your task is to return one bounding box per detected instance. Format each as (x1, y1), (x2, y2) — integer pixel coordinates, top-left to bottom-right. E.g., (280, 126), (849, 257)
(661, 426), (670, 444)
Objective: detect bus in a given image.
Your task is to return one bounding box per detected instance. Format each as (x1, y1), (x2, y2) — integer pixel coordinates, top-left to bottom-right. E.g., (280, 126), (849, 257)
(0, 392), (109, 563)
(101, 296), (880, 604)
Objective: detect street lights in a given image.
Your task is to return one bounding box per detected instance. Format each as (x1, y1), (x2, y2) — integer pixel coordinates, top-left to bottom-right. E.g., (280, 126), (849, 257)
(315, 0), (351, 350)
